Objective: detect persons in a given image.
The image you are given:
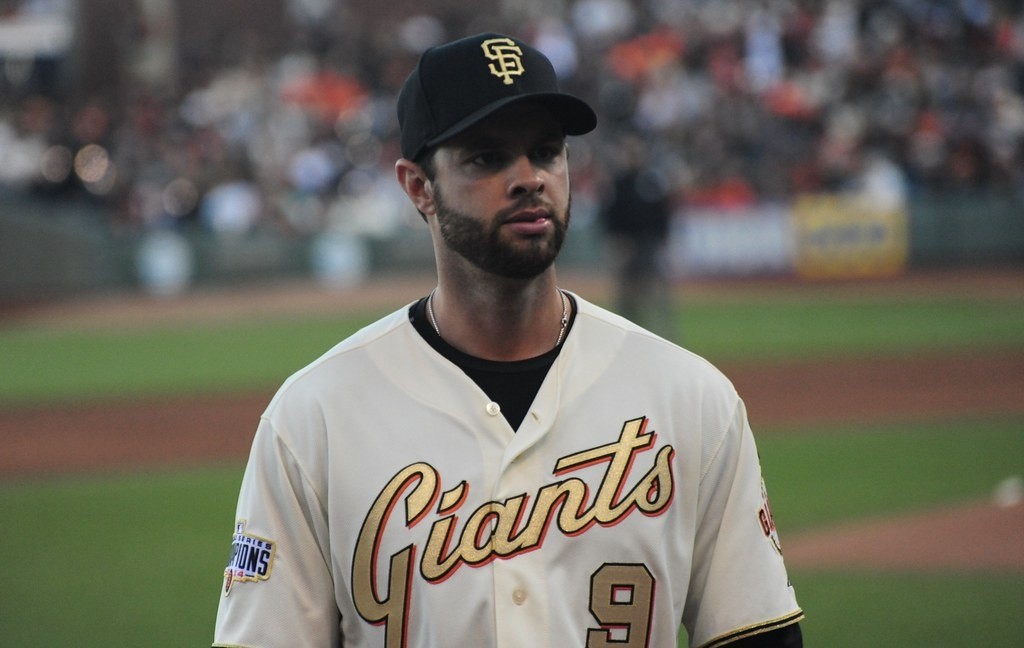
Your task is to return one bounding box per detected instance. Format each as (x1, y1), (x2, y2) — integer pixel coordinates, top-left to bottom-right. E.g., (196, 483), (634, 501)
(1, 0), (1024, 333)
(209, 32), (806, 648)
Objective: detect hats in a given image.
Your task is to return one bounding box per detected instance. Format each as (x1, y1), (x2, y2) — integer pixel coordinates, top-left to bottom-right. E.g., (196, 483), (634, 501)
(396, 30), (597, 161)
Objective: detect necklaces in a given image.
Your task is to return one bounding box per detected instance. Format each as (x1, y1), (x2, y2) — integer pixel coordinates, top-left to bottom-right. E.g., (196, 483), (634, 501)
(429, 285), (567, 347)
